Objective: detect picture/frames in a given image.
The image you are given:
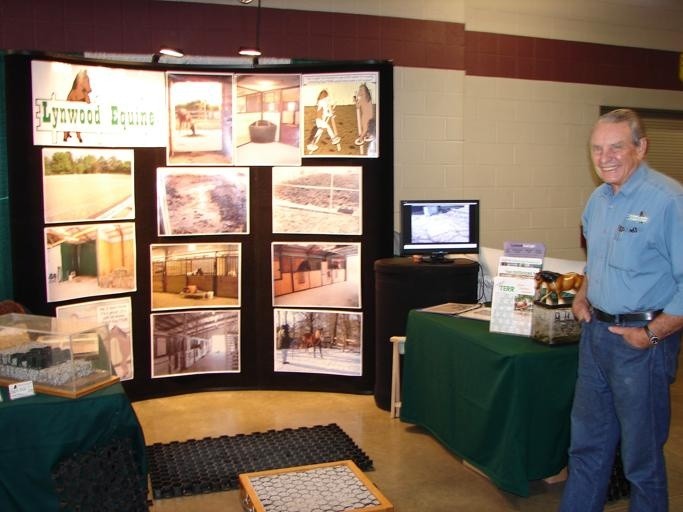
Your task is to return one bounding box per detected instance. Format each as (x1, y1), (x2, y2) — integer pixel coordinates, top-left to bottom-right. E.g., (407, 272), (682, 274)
(0, 329), (151, 510)
(0, 372), (120, 400)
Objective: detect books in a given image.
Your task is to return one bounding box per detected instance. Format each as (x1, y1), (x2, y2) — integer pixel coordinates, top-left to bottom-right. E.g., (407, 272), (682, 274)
(489, 258), (543, 337)
(417, 302), (491, 321)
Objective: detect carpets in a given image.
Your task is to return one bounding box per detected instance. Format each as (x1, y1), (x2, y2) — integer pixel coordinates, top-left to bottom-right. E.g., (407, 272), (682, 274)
(147, 421), (377, 503)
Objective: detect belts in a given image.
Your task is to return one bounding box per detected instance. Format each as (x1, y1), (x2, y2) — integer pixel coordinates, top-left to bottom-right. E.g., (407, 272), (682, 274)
(593, 307), (664, 323)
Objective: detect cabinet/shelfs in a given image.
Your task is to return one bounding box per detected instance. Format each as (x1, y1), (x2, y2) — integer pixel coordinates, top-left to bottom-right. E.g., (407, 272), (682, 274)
(236, 457), (396, 512)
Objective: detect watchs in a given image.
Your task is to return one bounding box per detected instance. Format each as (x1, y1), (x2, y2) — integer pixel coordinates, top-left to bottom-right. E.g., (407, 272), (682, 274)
(641, 325), (660, 347)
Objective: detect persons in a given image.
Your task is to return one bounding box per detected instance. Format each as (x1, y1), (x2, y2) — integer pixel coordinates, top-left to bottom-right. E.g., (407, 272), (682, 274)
(282, 325), (293, 364)
(558, 109), (683, 511)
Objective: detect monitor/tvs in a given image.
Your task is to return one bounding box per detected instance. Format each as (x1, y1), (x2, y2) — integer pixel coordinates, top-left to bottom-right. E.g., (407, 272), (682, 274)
(399, 200), (480, 263)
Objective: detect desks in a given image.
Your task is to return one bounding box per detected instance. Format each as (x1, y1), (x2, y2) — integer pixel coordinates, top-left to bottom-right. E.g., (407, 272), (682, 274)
(371, 256), (481, 414)
(398, 302), (579, 500)
